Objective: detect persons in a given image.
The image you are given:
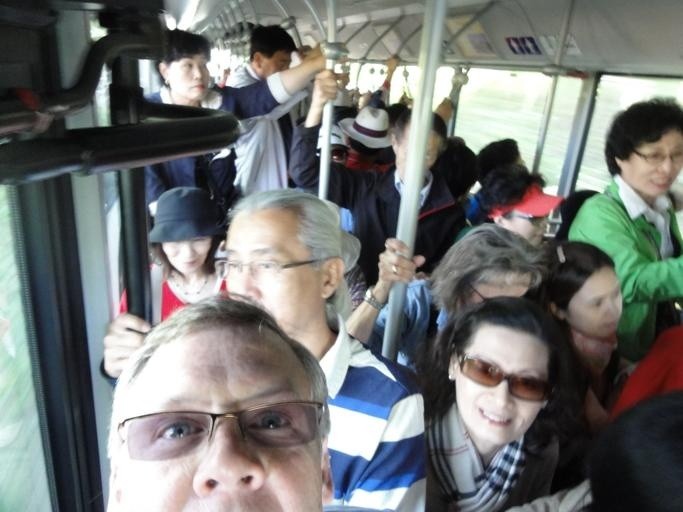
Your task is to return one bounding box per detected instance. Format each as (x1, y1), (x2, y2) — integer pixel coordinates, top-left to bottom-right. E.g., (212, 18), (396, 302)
(540, 96), (682, 434)
(592, 387), (682, 512)
(145, 25), (469, 320)
(118, 188), (228, 327)
(345, 140), (565, 375)
(101, 294), (332, 512)
(419, 296), (592, 512)
(100, 188), (427, 512)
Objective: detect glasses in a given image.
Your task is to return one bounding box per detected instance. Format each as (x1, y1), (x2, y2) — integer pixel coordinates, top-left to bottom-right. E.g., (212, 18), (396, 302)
(118, 401), (322, 460)
(457, 348), (555, 401)
(633, 149), (683, 163)
(317, 150), (343, 160)
(215, 260), (316, 280)
(515, 215), (542, 225)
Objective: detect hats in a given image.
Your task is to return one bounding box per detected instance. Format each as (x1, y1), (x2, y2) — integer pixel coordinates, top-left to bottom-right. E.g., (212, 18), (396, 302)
(316, 106), (392, 149)
(149, 187), (226, 243)
(488, 184), (565, 220)
